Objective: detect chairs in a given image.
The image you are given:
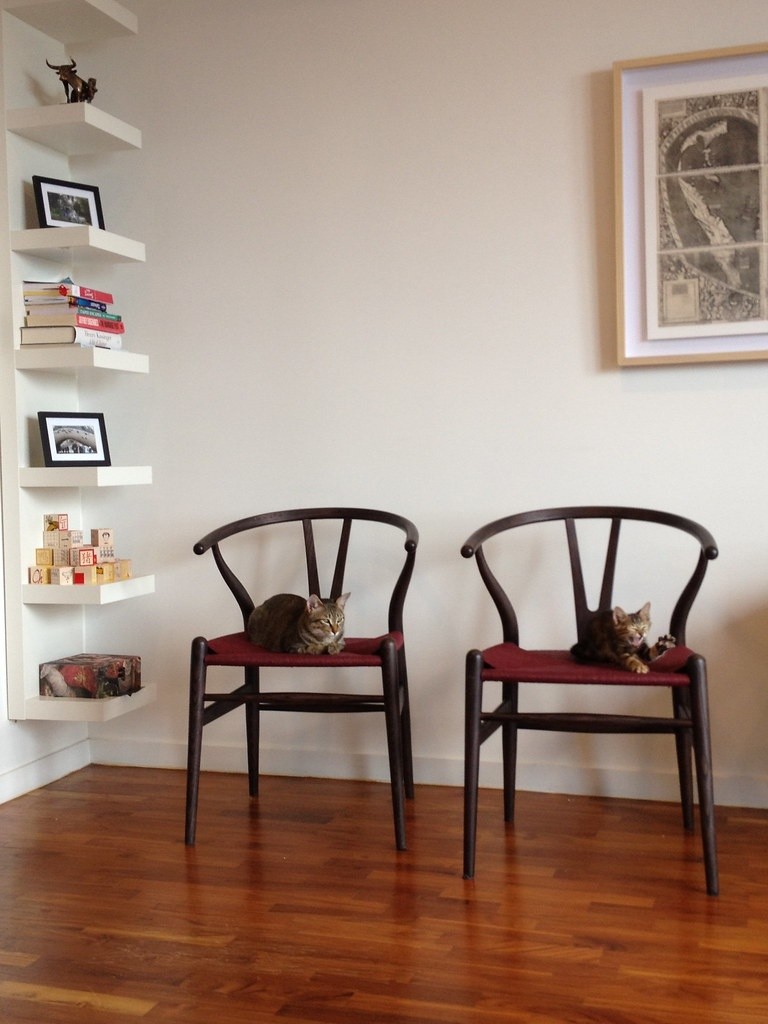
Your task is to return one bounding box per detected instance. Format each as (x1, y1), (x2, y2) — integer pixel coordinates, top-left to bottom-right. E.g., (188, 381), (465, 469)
(184, 507), (419, 851)
(461, 506), (721, 899)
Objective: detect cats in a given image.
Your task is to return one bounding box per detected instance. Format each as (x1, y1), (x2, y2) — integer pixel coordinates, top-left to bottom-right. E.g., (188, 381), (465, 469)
(570, 602), (677, 674)
(247, 593), (351, 654)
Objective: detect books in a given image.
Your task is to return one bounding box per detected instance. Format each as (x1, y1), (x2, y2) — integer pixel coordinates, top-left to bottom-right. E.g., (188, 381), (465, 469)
(19, 276), (125, 350)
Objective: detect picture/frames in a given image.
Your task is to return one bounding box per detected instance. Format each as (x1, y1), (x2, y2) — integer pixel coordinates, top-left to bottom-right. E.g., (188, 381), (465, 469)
(612, 40), (768, 367)
(32, 175), (106, 231)
(38, 412), (112, 467)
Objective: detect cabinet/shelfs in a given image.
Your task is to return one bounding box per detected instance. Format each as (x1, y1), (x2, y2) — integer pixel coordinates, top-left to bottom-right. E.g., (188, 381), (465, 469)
(0, 0), (157, 722)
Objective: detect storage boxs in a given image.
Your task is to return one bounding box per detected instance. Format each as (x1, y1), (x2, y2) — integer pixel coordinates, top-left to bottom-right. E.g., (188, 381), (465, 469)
(38, 653), (141, 699)
